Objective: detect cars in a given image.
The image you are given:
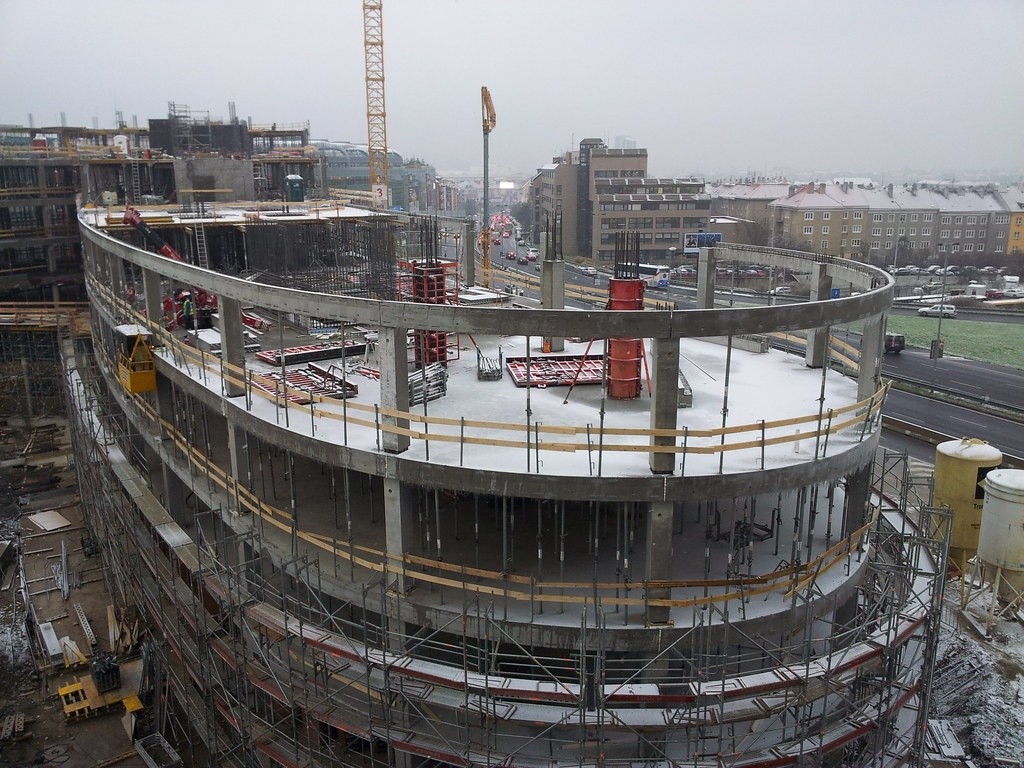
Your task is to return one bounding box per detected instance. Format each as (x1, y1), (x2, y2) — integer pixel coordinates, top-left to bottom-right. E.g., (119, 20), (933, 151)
(490, 214), (526, 246)
(918, 304), (957, 318)
(517, 257), (529, 265)
(884, 264), (1011, 276)
(670, 264), (770, 280)
(527, 248), (540, 261)
(913, 281), (1024, 300)
(581, 267), (597, 276)
(507, 252), (517, 260)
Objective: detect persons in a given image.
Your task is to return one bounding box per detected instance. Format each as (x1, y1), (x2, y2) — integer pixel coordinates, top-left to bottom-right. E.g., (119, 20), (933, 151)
(930, 278), (933, 282)
(687, 235), (696, 245)
(183, 295), (194, 330)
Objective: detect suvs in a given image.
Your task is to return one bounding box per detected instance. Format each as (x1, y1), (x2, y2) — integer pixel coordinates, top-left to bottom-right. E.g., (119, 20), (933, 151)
(885, 332), (905, 354)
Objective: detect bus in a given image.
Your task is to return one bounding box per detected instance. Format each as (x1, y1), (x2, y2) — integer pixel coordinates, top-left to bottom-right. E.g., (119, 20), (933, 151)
(614, 262), (670, 288)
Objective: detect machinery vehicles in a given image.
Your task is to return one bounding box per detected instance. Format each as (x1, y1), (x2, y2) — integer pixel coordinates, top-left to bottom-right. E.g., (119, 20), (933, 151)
(122, 201), (219, 331)
